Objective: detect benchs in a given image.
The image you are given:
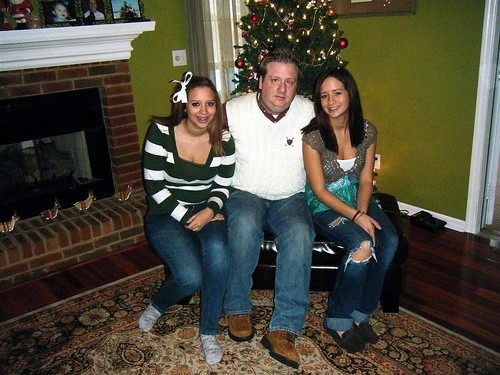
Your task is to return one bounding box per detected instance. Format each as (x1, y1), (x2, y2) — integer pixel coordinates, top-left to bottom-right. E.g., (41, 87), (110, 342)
(176, 188), (411, 315)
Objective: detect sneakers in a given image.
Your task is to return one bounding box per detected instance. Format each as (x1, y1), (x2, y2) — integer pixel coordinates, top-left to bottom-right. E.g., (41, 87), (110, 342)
(227, 314), (255, 343)
(260, 330), (301, 369)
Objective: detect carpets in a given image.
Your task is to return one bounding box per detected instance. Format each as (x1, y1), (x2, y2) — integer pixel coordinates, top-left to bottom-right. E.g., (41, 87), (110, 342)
(0, 262), (500, 375)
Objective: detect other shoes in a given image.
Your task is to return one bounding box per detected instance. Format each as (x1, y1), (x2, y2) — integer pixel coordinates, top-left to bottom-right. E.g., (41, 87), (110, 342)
(322, 320), (366, 351)
(353, 321), (379, 344)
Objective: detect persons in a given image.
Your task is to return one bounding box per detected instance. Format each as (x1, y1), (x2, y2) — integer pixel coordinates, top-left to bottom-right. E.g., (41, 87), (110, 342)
(301, 68), (399, 354)
(84, 0), (105, 20)
(51, 1), (72, 21)
(139, 76), (236, 364)
(6, 0), (33, 29)
(222, 49), (317, 369)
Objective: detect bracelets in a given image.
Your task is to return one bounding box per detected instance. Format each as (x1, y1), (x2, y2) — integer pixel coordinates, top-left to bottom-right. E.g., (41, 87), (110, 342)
(352, 210), (361, 223)
